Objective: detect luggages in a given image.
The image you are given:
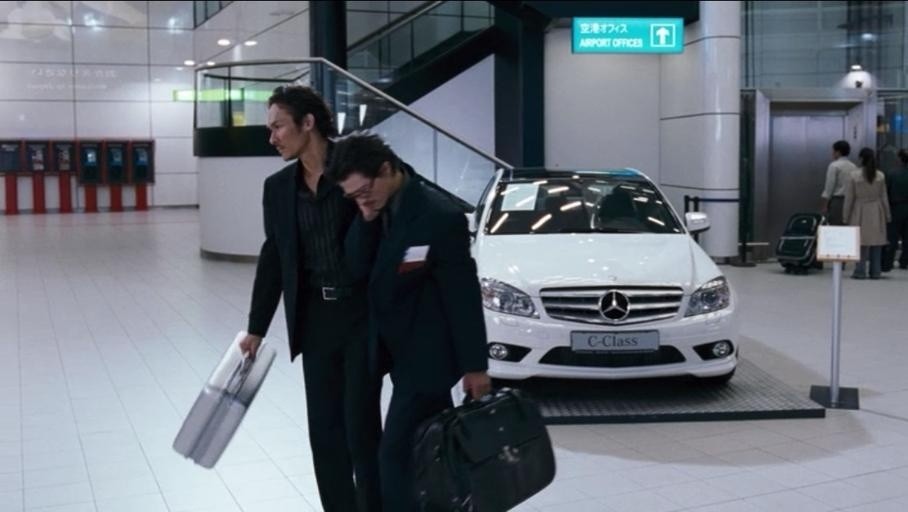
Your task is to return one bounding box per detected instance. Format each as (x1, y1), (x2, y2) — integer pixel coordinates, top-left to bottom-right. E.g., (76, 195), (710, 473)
(776, 211), (827, 274)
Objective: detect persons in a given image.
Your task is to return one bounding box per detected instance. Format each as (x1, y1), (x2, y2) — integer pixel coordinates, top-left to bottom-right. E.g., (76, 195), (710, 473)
(240, 84), (385, 512)
(323, 134), (492, 512)
(820, 113), (908, 281)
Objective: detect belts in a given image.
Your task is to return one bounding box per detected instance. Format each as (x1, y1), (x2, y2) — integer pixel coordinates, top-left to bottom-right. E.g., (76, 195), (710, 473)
(312, 286), (350, 302)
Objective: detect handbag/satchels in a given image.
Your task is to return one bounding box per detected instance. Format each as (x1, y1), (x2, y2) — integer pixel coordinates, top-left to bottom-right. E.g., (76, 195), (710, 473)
(411, 388), (556, 512)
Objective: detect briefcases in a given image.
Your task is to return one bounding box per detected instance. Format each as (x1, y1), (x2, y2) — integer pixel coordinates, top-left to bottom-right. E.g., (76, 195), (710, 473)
(171, 329), (277, 470)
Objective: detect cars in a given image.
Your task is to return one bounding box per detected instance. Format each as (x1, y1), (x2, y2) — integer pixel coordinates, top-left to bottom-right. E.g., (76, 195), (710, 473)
(469, 167), (738, 391)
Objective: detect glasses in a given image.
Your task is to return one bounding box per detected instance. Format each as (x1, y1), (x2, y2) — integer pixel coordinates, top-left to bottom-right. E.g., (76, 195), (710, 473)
(343, 176), (375, 199)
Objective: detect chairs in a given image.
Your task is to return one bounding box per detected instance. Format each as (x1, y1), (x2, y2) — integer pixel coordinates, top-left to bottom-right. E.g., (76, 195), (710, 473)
(599, 191), (638, 218)
